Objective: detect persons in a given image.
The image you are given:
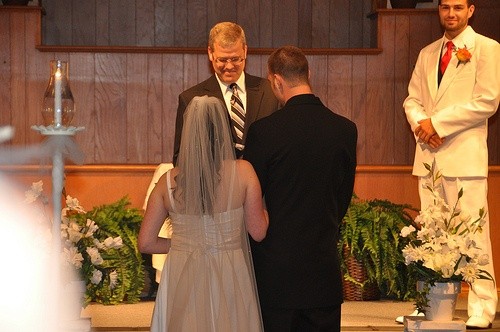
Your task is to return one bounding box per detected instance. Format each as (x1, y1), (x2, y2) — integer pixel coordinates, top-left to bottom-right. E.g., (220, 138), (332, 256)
(396, 0), (500, 328)
(138, 95), (269, 331)
(171, 21), (278, 167)
(243, 45), (357, 332)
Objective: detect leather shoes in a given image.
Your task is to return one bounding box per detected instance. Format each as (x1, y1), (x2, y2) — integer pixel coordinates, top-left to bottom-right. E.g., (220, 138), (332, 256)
(465, 315), (492, 329)
(395, 307), (425, 324)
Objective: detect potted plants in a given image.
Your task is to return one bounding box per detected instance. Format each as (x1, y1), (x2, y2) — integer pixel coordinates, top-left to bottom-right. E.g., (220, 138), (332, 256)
(399, 157), (495, 332)
(23, 173), (154, 304)
(336, 191), (422, 301)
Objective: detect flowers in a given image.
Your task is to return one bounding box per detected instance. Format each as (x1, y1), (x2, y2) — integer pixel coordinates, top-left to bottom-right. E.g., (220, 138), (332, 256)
(453, 43), (473, 68)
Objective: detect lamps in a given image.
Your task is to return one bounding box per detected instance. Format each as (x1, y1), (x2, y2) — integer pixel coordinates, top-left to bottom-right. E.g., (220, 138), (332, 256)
(53, 59), (63, 126)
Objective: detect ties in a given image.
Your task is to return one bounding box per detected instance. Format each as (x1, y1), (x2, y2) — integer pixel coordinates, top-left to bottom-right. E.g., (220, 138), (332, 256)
(440, 41), (454, 76)
(230, 83), (245, 150)
